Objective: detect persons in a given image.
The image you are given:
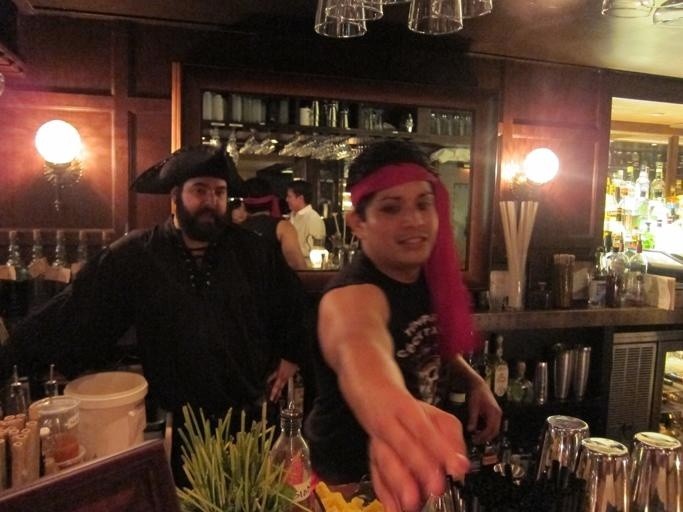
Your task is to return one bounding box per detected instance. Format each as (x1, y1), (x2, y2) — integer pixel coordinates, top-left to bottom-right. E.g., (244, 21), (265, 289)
(53, 145), (309, 488)
(240, 178), (307, 269)
(285, 180), (327, 258)
(300, 140), (503, 511)
(227, 199), (248, 226)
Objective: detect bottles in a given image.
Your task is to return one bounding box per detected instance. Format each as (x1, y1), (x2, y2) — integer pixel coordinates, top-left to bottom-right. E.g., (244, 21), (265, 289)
(0, 377), (70, 496)
(468, 420), (534, 483)
(0, 229), (117, 317)
(659, 370), (683, 438)
(593, 237), (628, 304)
(448, 333), (534, 404)
(625, 236), (647, 306)
(269, 395), (314, 512)
(201, 91), (472, 137)
(606, 143), (682, 252)
(530, 281), (553, 310)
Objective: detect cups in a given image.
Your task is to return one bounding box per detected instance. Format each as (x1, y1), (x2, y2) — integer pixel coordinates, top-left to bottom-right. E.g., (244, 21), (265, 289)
(629, 430), (682, 511)
(487, 272), (527, 312)
(571, 263), (592, 303)
(531, 341), (592, 402)
(602, 1), (683, 30)
(574, 431), (629, 512)
(314, 1), (493, 38)
(535, 414), (588, 482)
(28, 394), (85, 468)
(552, 261), (571, 308)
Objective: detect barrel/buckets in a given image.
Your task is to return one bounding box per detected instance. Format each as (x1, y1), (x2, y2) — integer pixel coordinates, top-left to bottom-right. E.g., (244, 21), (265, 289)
(64, 370), (150, 459)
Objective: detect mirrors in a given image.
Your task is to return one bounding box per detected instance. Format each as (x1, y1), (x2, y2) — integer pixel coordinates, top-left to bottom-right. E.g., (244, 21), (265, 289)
(170, 61), (501, 294)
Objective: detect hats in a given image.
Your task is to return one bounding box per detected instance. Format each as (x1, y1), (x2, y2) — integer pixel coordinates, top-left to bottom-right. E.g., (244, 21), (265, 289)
(129, 143), (251, 195)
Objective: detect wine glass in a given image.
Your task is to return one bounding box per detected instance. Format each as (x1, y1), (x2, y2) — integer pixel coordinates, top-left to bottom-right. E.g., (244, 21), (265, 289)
(206, 126), (367, 167)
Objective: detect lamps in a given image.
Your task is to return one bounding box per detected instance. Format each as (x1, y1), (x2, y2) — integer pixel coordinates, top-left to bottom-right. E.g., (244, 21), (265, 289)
(511, 147), (559, 204)
(35, 119), (83, 213)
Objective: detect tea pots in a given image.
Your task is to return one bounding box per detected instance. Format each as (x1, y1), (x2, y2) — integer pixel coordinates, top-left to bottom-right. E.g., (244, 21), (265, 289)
(305, 232), (327, 271)
